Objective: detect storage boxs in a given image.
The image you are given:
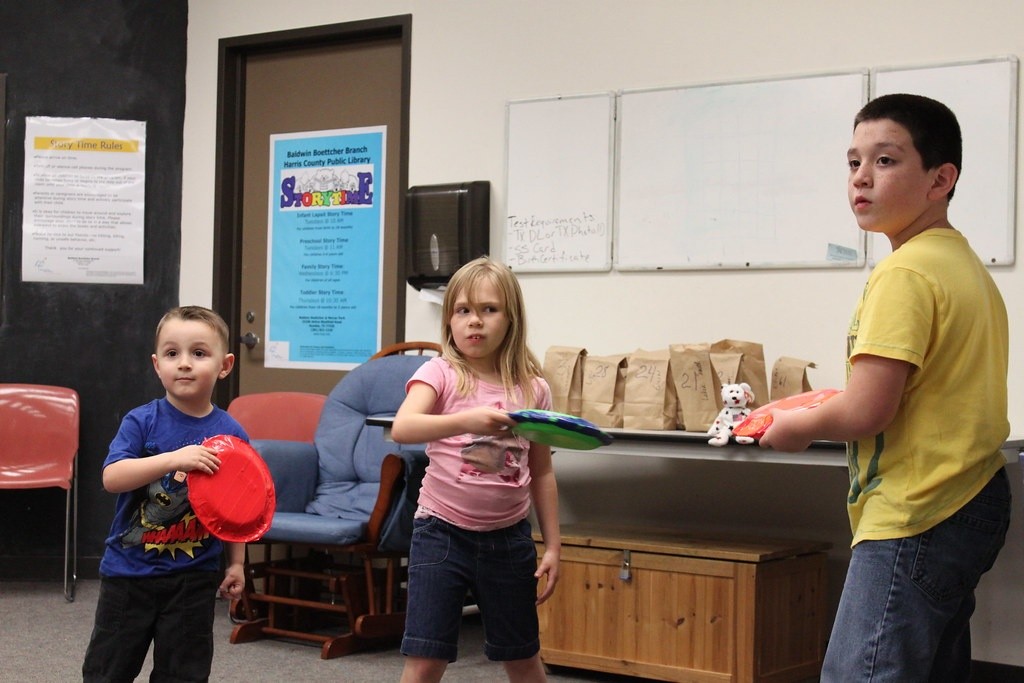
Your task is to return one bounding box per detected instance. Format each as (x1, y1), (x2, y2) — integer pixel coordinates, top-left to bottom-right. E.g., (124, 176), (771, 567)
(533, 521), (836, 683)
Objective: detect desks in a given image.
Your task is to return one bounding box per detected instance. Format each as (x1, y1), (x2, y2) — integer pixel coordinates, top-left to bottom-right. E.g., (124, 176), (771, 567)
(365, 414), (1024, 467)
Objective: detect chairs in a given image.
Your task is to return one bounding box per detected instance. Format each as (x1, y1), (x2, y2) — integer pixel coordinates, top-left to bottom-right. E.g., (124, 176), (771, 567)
(226, 342), (443, 659)
(0, 384), (80, 602)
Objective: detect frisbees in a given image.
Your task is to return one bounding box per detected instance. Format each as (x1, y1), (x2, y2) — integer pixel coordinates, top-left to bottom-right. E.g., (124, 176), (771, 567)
(732, 387), (840, 441)
(506, 408), (616, 451)
(187, 432), (278, 543)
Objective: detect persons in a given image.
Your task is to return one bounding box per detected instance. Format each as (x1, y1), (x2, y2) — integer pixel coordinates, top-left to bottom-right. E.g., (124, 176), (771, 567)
(757, 93), (1014, 683)
(390, 253), (563, 683)
(80, 304), (247, 683)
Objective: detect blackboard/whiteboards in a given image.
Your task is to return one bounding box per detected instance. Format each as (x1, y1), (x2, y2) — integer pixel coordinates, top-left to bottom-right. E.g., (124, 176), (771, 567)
(500, 53), (1019, 274)
